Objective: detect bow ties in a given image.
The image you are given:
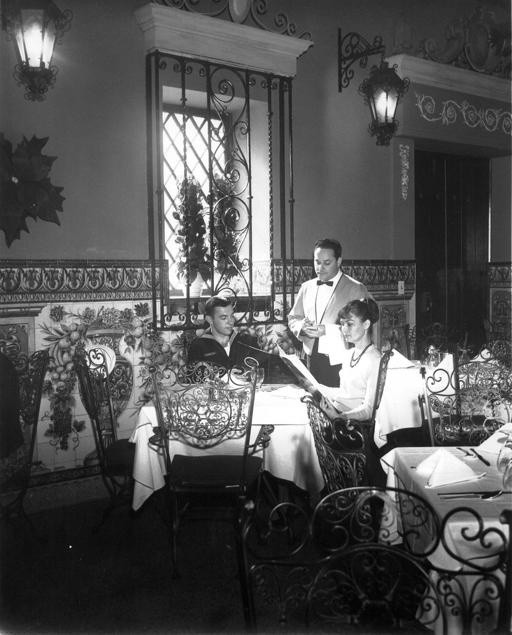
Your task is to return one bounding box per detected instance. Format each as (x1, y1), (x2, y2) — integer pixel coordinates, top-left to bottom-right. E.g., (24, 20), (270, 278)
(317, 280), (332, 286)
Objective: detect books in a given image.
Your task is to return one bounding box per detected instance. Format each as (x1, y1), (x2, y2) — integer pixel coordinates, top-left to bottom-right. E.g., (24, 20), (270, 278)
(276, 343), (320, 391)
(234, 339), (307, 385)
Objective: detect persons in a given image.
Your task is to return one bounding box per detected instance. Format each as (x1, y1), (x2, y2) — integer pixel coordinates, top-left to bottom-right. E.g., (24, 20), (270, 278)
(310, 297), (385, 422)
(184, 295), (262, 386)
(287, 236), (370, 388)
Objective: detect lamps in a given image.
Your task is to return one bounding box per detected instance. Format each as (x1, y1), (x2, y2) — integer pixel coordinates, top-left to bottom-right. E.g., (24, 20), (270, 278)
(334, 27), (407, 147)
(3, 1), (72, 103)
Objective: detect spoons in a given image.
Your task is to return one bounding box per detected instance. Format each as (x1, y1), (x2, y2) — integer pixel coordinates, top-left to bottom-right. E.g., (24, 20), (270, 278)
(456, 447), (473, 457)
(438, 490), (503, 502)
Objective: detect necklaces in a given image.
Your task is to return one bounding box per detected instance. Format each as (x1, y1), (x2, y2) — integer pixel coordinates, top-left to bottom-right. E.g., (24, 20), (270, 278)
(350, 340), (375, 368)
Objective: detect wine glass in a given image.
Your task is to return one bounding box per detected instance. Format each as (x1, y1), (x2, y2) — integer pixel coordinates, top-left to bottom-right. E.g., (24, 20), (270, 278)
(496, 430), (512, 492)
(250, 367), (264, 395)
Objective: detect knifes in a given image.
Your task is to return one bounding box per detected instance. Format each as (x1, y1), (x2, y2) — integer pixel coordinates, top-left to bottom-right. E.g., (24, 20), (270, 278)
(437, 490), (512, 496)
(470, 448), (490, 466)
